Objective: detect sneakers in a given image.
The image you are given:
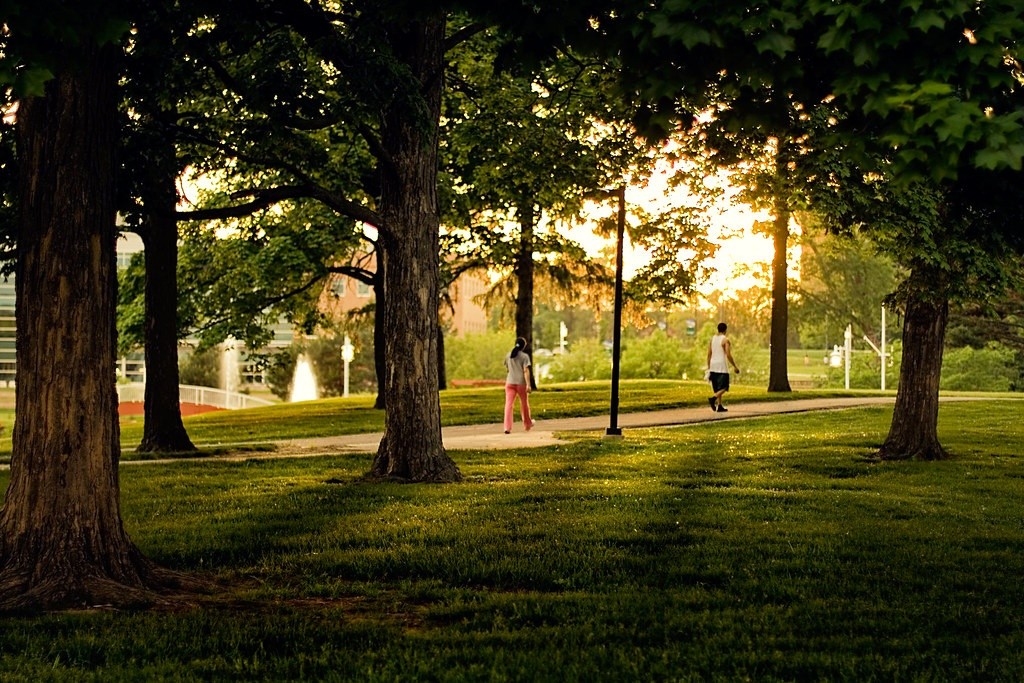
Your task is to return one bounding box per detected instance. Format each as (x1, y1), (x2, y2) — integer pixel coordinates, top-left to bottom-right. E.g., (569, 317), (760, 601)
(717, 404), (729, 412)
(524, 418), (536, 432)
(503, 429), (511, 434)
(708, 396), (717, 412)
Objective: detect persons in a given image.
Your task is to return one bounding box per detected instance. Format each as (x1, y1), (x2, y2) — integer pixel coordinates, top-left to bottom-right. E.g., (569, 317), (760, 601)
(706, 322), (740, 412)
(501, 337), (535, 434)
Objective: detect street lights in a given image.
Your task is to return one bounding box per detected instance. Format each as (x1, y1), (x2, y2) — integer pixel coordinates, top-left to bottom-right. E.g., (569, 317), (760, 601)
(584, 181), (628, 436)
